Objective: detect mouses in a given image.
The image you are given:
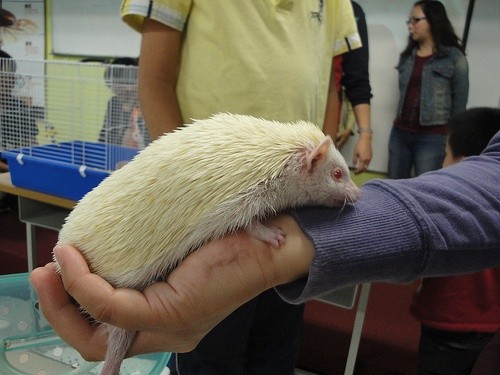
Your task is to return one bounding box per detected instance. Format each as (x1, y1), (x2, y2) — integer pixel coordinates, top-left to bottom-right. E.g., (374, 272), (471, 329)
(50, 112), (361, 375)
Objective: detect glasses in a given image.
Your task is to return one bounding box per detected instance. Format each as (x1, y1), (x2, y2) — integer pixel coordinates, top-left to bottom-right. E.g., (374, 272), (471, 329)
(406, 15), (427, 25)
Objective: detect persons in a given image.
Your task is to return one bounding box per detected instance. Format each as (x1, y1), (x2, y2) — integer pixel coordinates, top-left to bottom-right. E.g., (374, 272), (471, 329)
(32, 131), (500, 363)
(387, 0), (470, 179)
(98, 58), (153, 150)
(440, 106), (500, 172)
(0, 52), (40, 151)
(119, 1), (374, 176)
(322, 1), (373, 174)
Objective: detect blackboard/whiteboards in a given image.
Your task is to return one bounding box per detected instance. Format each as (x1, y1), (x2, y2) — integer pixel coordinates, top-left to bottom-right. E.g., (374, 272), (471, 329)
(52, 0), (149, 59)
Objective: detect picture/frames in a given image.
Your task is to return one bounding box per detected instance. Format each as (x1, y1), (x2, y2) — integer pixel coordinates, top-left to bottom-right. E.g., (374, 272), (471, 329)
(0, 0), (47, 120)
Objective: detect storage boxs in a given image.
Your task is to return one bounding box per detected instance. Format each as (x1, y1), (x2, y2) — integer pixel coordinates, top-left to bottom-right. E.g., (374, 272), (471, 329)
(3, 138), (140, 205)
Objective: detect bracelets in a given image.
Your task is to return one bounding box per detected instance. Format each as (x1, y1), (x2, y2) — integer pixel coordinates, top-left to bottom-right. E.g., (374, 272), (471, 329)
(357, 127), (371, 133)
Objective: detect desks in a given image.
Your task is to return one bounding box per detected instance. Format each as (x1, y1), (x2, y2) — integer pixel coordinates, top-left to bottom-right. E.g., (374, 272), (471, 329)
(0, 129), (422, 375)
